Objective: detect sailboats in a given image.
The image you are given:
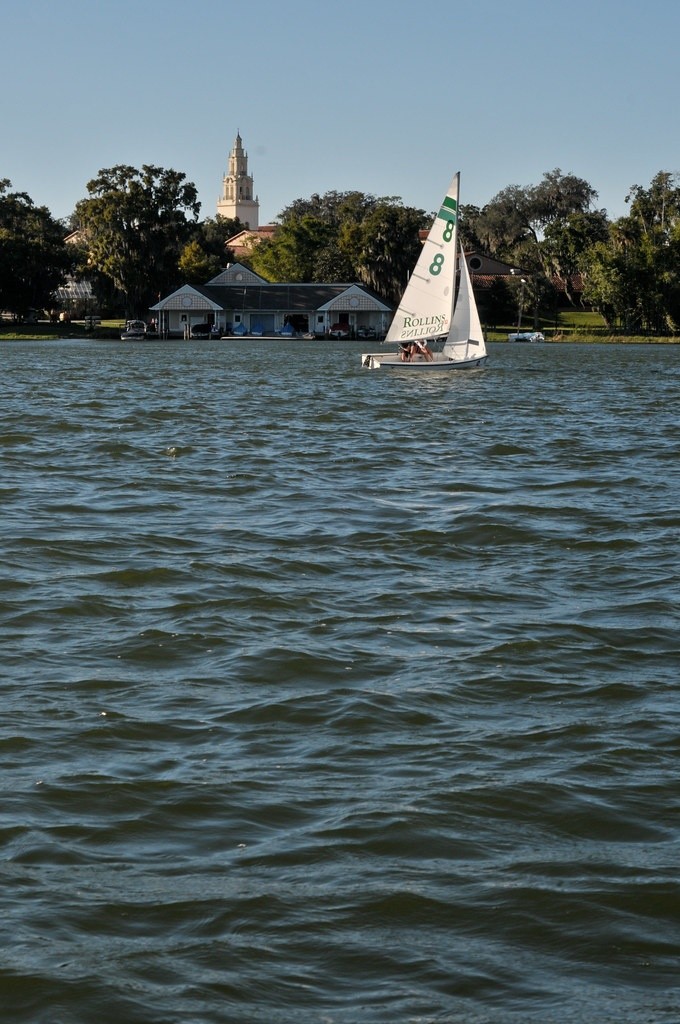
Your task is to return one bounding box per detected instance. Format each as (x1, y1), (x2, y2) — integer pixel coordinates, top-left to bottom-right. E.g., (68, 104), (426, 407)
(360, 171), (489, 371)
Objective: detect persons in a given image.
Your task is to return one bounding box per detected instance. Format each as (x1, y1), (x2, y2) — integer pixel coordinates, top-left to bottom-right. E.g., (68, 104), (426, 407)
(414, 339), (434, 362)
(398, 342), (415, 362)
